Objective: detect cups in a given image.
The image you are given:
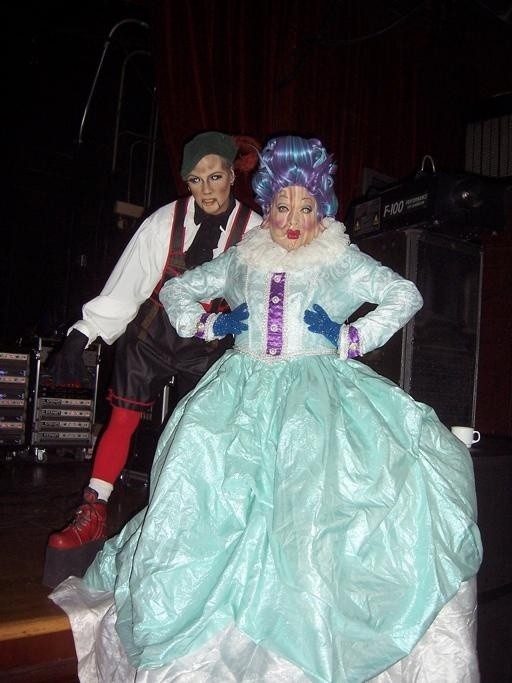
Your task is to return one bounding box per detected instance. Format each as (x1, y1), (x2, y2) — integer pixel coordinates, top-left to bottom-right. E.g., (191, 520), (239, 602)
(452, 426), (481, 448)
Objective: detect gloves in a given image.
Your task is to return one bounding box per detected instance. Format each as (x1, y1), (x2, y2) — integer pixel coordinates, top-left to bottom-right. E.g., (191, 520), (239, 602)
(44, 327), (90, 385)
(303, 303), (348, 359)
(204, 303), (250, 342)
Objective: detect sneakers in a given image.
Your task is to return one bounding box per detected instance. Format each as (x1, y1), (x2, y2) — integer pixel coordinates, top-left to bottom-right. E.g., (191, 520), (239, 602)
(47, 487), (108, 550)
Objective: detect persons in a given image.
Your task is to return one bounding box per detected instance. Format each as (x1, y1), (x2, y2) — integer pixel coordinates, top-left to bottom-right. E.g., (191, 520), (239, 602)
(44, 125), (270, 554)
(158, 131), (428, 683)
